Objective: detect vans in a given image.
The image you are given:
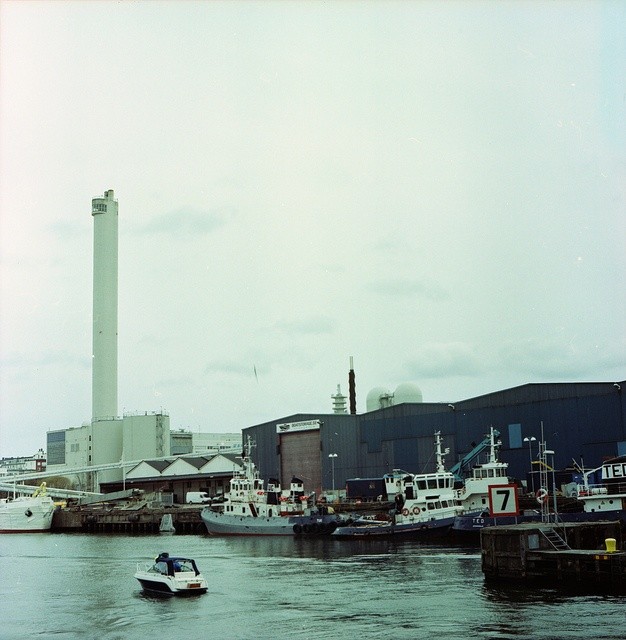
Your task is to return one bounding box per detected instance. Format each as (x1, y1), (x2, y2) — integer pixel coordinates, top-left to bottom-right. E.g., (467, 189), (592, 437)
(185, 492), (211, 505)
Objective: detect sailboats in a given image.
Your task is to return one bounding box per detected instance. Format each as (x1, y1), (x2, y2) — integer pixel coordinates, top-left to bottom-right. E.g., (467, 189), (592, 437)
(452, 421), (625, 531)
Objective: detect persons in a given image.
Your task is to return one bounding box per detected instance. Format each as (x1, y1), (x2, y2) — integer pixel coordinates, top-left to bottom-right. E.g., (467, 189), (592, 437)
(156, 552), (175, 577)
(394, 490), (403, 515)
(316, 494), (326, 516)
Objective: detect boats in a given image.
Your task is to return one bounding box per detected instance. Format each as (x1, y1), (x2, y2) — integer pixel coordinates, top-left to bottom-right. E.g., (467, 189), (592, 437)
(0, 481), (55, 535)
(133, 552), (209, 595)
(199, 430), (417, 537)
(328, 430), (521, 538)
(519, 436), (579, 506)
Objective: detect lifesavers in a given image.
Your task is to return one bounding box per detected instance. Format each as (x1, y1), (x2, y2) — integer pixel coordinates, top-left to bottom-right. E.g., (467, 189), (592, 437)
(413, 507), (420, 515)
(536, 489), (548, 504)
(402, 508), (409, 516)
